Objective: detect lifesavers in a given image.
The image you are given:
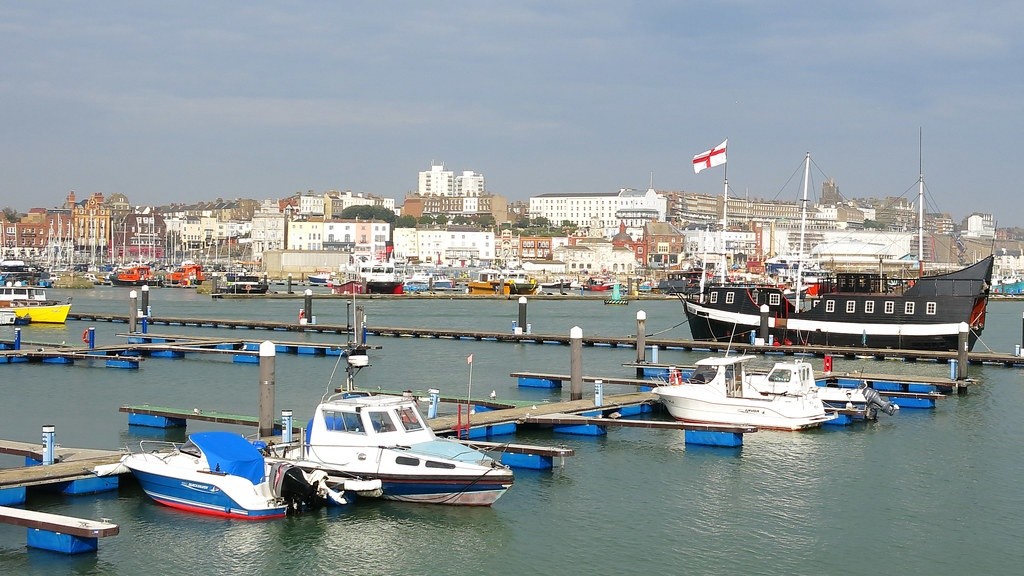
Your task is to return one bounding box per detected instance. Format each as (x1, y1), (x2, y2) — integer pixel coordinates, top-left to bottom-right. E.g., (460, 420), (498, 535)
(82, 329), (90, 344)
(299, 311), (305, 320)
(670, 369), (682, 386)
(246, 284), (251, 290)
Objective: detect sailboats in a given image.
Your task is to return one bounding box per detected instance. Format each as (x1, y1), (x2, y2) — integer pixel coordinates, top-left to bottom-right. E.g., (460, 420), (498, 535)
(0, 215), (267, 325)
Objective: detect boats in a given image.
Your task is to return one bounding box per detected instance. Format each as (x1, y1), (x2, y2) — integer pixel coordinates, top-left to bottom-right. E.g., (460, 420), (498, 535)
(307, 247), (539, 296)
(671, 126), (995, 353)
(123, 428), (318, 521)
(263, 341), (514, 508)
(649, 353), (839, 433)
(583, 246), (831, 295)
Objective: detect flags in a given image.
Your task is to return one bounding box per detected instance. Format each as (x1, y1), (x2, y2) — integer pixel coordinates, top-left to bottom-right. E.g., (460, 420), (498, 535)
(693, 140), (726, 173)
